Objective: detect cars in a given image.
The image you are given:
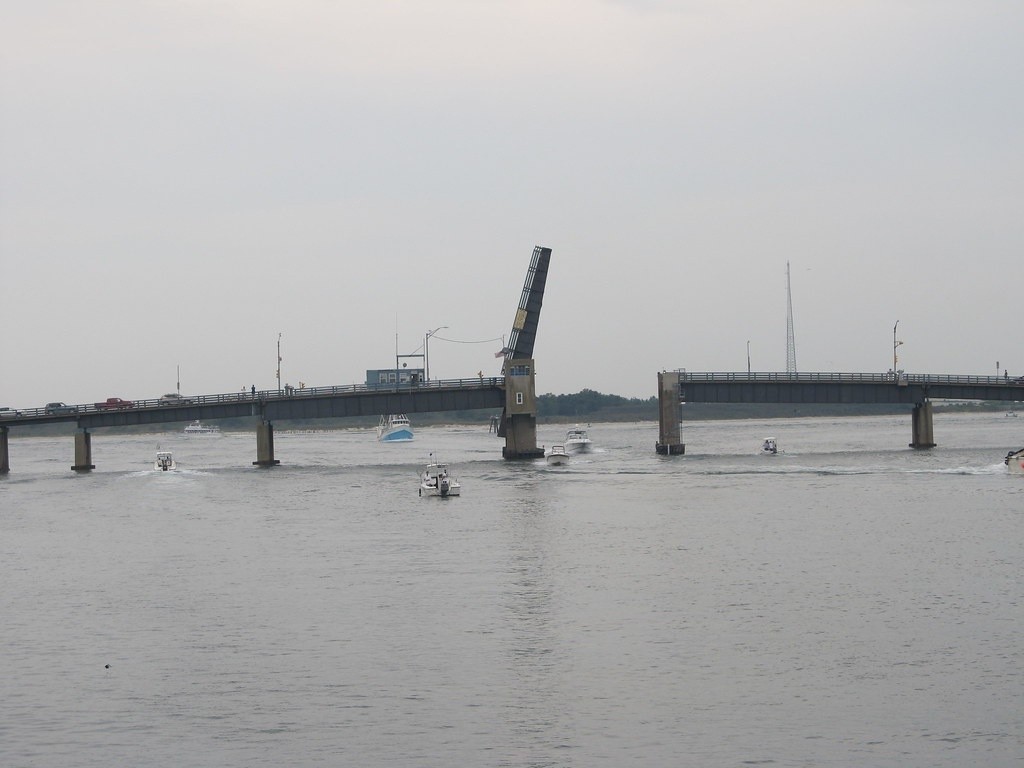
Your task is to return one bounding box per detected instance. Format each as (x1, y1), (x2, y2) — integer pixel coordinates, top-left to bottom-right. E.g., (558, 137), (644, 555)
(0, 407), (26, 419)
(159, 394), (193, 406)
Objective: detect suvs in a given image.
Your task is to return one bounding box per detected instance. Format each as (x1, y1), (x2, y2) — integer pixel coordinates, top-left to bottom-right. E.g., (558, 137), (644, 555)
(45, 402), (77, 415)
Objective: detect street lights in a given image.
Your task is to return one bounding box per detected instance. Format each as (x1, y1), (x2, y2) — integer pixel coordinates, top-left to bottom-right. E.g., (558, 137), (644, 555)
(426, 327), (447, 386)
(894, 320), (903, 372)
(747, 341), (751, 379)
(278, 333), (282, 394)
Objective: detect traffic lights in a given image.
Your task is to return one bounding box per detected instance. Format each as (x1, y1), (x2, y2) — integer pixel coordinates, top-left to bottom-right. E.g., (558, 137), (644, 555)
(276, 369), (279, 378)
(895, 356), (897, 362)
(302, 383), (305, 388)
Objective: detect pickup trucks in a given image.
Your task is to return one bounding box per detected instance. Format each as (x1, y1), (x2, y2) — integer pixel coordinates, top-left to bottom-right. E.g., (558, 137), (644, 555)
(95, 398), (134, 410)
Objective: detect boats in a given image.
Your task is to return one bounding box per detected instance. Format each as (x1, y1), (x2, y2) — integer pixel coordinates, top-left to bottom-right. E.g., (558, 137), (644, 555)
(544, 446), (569, 465)
(760, 437), (780, 455)
(155, 452), (176, 472)
(184, 420), (222, 437)
(418, 452), (461, 497)
(376, 412), (414, 441)
(1005, 449), (1024, 474)
(1005, 412), (1017, 417)
(563, 424), (593, 455)
